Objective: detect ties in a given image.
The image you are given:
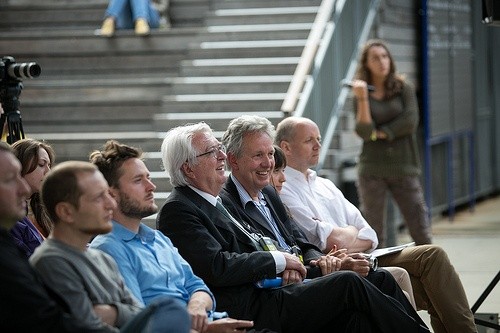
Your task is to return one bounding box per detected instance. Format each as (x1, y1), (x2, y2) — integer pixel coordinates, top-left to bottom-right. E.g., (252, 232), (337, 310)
(215, 199), (264, 251)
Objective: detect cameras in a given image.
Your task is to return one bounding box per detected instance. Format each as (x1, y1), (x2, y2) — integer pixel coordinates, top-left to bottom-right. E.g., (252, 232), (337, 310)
(0, 56), (41, 81)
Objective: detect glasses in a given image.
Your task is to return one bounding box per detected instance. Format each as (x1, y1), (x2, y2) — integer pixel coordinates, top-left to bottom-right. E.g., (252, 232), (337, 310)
(186, 143), (226, 163)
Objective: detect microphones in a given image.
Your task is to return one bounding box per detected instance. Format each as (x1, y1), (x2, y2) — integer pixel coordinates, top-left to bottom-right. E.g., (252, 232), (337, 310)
(341, 79), (376, 92)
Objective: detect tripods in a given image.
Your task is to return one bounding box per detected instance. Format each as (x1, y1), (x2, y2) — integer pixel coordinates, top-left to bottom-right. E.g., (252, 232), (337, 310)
(0, 81), (25, 145)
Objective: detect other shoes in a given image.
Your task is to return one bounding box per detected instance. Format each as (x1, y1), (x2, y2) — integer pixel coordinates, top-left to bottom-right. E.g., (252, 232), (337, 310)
(100, 18), (114, 37)
(135, 18), (150, 35)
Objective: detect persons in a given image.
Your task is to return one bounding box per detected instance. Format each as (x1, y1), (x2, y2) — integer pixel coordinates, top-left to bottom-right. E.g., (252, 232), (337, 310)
(89, 140), (254, 333)
(93, 0), (171, 37)
(10, 139), (56, 258)
(29, 161), (146, 333)
(0, 102), (27, 146)
(273, 116), (478, 333)
(156, 122), (431, 333)
(220, 115), (431, 333)
(269, 145), (417, 312)
(351, 39), (432, 250)
(0, 141), (108, 333)
